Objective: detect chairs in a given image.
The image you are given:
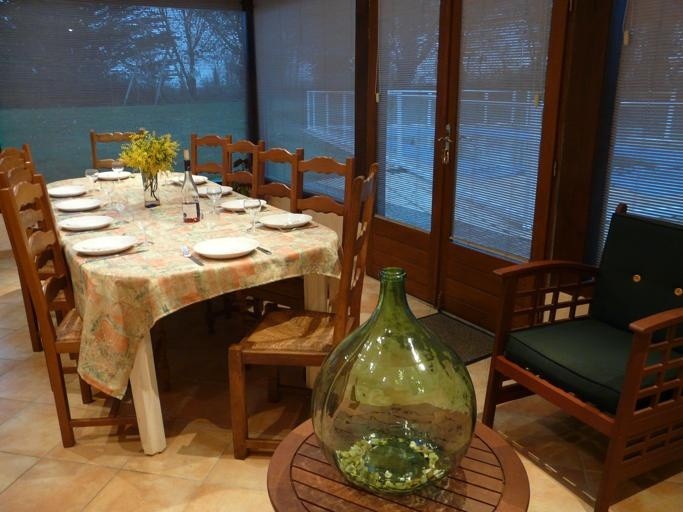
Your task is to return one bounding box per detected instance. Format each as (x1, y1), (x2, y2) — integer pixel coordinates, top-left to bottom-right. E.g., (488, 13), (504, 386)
(89, 127), (148, 173)
(240, 153), (356, 312)
(205, 135), (265, 330)
(1, 144), (173, 450)
(238, 143), (304, 342)
(483, 201), (682, 511)
(224, 163), (380, 454)
(190, 131), (232, 188)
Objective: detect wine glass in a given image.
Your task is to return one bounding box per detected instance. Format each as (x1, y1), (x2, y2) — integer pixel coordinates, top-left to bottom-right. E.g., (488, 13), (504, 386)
(83, 158), (153, 247)
(241, 199), (262, 235)
(206, 185), (222, 217)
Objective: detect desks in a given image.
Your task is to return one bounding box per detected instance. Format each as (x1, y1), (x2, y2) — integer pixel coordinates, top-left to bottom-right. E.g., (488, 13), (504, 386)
(45, 173), (341, 461)
(266, 401), (531, 510)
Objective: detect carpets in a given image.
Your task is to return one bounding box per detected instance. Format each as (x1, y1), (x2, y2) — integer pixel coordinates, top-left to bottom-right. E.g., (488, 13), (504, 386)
(417, 312), (493, 365)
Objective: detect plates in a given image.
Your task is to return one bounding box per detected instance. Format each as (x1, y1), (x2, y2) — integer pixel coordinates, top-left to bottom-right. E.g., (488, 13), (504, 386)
(196, 182), (232, 198)
(55, 197), (102, 212)
(221, 197), (264, 212)
(59, 214), (113, 231)
(171, 173), (207, 185)
(193, 236), (256, 260)
(72, 236), (140, 255)
(46, 186), (85, 198)
(259, 212), (312, 229)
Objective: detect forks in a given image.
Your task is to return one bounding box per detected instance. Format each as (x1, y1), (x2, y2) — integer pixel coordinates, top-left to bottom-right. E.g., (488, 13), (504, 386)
(179, 245), (204, 266)
(277, 225), (318, 232)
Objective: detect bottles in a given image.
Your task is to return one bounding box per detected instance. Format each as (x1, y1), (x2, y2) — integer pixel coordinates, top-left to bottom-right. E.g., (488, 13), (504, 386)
(180, 149), (199, 223)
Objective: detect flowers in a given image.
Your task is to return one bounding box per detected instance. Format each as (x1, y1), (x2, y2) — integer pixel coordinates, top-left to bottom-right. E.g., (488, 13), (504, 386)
(120, 133), (178, 208)
